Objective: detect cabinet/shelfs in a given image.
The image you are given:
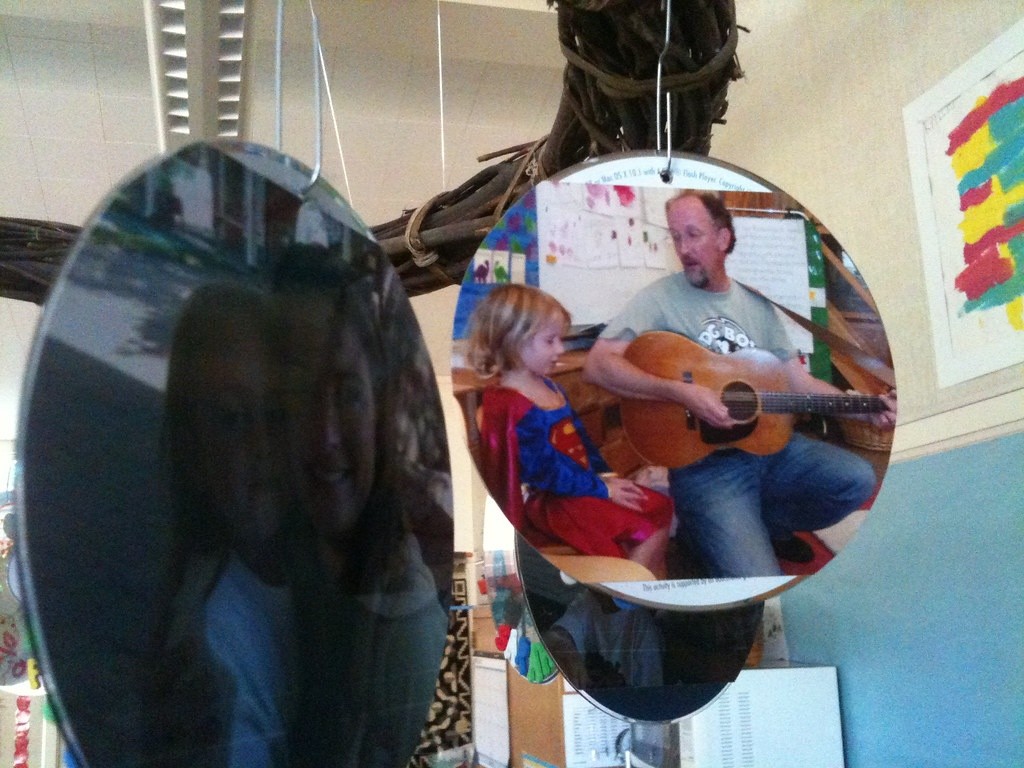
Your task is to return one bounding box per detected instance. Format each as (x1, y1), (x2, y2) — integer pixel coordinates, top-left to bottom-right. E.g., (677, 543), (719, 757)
(451, 346), (649, 482)
(473, 603), (563, 768)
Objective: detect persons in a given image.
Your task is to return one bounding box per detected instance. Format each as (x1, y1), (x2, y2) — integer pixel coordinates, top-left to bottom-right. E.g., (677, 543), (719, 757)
(468, 193), (897, 689)
(157, 244), (452, 768)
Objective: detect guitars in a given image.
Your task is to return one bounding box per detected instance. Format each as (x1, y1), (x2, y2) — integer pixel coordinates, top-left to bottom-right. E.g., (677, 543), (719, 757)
(618, 330), (895, 469)
